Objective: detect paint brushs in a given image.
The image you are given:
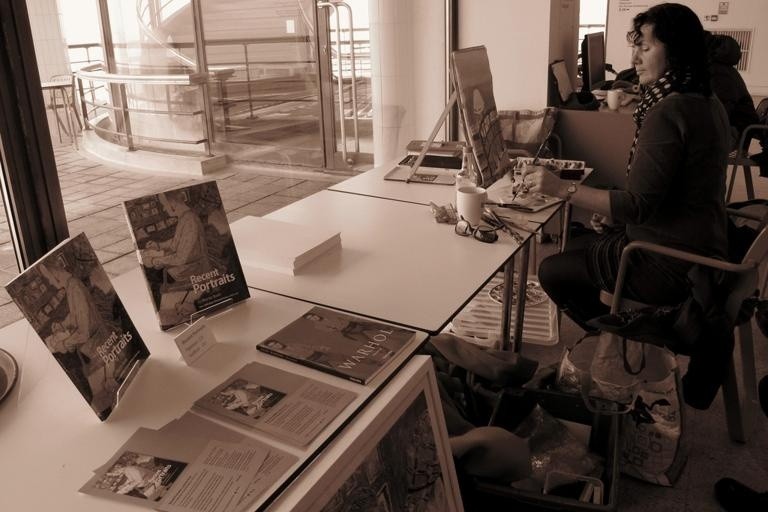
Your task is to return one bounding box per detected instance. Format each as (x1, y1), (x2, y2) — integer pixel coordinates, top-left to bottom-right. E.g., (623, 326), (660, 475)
(511, 128), (553, 201)
(481, 206), (541, 249)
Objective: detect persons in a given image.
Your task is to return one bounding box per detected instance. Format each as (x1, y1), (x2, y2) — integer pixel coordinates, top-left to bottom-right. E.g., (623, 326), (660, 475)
(221, 388), (260, 411)
(611, 29), (758, 150)
(264, 339), (383, 368)
(521, 3), (731, 411)
(39, 260), (118, 392)
(175, 201), (250, 314)
(144, 191), (212, 281)
(87, 255), (151, 391)
(714, 296), (768, 512)
(108, 463), (161, 494)
(303, 312), (410, 356)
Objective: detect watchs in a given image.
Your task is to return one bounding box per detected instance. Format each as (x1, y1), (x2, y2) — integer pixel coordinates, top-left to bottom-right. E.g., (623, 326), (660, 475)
(565, 182), (578, 202)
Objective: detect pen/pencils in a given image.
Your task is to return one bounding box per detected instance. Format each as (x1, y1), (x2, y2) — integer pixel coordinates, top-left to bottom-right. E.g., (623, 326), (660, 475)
(498, 203), (532, 211)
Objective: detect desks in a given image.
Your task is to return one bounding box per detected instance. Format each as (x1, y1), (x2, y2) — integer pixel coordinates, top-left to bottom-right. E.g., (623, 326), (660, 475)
(240, 190), (544, 352)
(550, 102), (637, 190)
(327, 158), (597, 353)
(40, 81), (80, 152)
(0, 264), (432, 511)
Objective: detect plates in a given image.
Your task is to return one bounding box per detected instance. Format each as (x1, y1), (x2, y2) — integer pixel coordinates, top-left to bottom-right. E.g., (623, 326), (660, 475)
(0, 347), (18, 404)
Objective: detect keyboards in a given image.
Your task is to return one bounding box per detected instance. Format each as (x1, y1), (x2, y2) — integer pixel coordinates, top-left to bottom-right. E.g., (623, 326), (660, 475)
(620, 93), (633, 105)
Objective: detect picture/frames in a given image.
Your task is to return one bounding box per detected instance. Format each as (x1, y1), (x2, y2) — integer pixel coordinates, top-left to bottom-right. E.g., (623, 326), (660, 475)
(260, 353), (467, 511)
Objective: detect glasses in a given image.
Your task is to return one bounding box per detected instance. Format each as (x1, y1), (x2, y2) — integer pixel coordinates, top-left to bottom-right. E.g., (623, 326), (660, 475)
(454, 214), (499, 243)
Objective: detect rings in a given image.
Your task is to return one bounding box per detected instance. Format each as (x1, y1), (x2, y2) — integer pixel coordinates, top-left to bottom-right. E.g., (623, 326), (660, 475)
(529, 179), (533, 187)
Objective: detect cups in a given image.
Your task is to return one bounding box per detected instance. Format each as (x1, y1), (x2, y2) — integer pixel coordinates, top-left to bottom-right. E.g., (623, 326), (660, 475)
(607, 89), (623, 111)
(458, 188), (489, 226)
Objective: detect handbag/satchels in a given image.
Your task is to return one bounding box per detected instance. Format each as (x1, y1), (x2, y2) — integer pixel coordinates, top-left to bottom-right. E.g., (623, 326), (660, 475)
(497, 107), (560, 159)
(556, 332), (690, 488)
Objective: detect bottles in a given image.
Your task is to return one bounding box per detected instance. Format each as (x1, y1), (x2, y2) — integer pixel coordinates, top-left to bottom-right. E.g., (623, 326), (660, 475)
(455, 143), (479, 191)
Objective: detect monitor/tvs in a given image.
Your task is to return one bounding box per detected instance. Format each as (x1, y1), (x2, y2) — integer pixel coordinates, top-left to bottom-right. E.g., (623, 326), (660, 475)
(582, 31), (606, 100)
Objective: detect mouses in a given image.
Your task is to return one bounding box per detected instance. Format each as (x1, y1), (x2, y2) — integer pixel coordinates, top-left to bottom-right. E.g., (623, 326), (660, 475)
(615, 88), (624, 93)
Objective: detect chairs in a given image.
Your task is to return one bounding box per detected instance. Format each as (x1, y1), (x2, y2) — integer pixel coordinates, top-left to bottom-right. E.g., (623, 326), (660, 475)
(46, 73), (85, 130)
(726, 92), (766, 205)
(591, 206), (767, 444)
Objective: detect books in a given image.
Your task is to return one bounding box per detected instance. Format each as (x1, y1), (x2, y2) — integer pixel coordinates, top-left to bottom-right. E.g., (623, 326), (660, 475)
(256, 304), (417, 386)
(4, 231), (151, 420)
(120, 179), (250, 331)
(229, 214), (342, 277)
